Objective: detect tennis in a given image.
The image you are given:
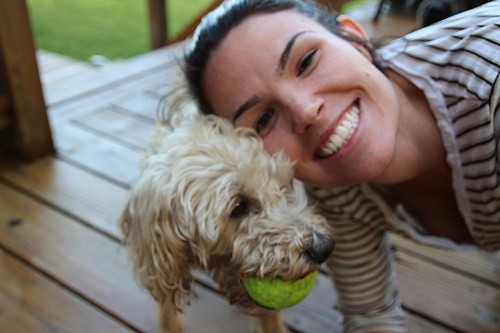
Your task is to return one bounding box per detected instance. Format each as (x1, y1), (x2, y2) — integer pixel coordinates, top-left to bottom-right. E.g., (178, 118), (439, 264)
(244, 270), (317, 310)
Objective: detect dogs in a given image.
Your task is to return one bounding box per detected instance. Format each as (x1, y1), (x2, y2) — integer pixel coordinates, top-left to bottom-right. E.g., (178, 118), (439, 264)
(117, 91), (335, 333)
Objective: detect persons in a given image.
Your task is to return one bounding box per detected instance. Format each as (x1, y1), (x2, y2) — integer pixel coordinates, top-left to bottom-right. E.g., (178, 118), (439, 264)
(179, 0), (500, 333)
